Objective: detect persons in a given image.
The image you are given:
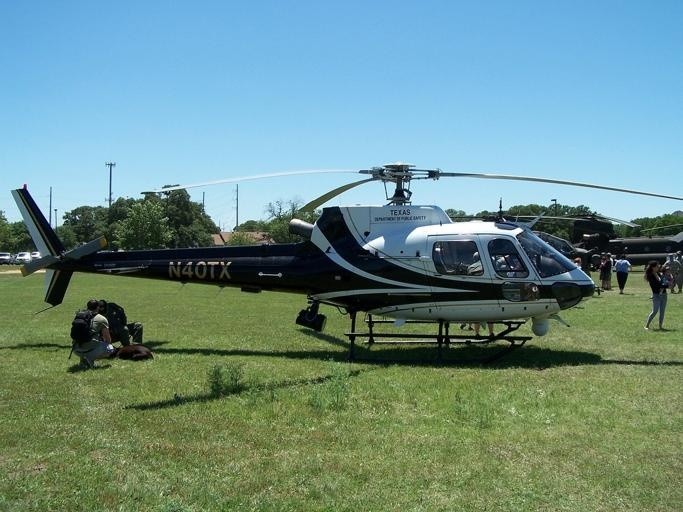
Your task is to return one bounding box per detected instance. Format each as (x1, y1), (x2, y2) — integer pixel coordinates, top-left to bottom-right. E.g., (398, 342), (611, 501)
(674, 251), (683, 294)
(573, 258), (582, 270)
(613, 254), (631, 294)
(660, 252), (683, 294)
(467, 251), (495, 337)
(599, 252), (611, 290)
(98, 299), (143, 347)
(460, 323), (473, 332)
(649, 265), (674, 300)
(600, 252), (613, 289)
(642, 260), (674, 331)
(71, 299), (113, 370)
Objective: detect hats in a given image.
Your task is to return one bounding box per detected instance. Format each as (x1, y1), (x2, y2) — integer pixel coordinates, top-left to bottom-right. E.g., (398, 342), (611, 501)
(98, 300), (107, 315)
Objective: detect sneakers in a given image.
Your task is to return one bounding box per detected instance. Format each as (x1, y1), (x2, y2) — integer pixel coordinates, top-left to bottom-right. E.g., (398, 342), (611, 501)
(82, 356), (93, 368)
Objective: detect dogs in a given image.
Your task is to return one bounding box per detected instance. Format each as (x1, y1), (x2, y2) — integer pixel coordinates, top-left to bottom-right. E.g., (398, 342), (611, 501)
(108, 343), (153, 361)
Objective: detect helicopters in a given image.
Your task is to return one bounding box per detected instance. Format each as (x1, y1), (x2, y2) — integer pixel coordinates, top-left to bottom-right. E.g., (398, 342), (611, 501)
(8, 161), (683, 367)
(449, 208), (683, 274)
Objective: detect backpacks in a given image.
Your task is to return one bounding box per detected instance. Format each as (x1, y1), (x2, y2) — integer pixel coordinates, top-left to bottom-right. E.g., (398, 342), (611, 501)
(71, 310), (99, 343)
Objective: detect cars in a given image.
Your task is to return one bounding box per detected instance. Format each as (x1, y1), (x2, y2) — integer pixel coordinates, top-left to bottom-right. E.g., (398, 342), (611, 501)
(0, 251), (43, 266)
